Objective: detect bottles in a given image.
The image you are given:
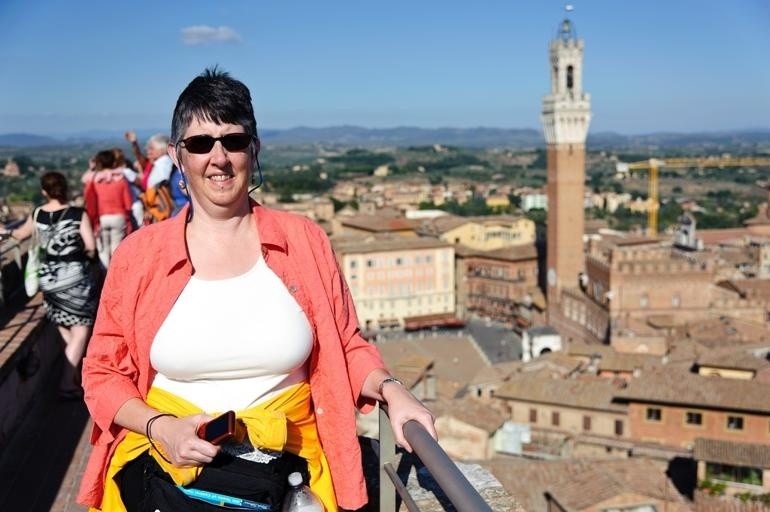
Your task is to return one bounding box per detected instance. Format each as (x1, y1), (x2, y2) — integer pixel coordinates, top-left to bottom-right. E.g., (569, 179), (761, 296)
(284, 471), (326, 512)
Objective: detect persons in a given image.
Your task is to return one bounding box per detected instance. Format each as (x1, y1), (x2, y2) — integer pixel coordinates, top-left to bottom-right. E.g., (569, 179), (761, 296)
(33, 129), (192, 399)
(71, 65), (439, 512)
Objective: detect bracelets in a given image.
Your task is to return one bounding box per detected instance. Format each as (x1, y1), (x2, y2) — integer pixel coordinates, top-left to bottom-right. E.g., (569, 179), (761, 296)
(379, 378), (403, 399)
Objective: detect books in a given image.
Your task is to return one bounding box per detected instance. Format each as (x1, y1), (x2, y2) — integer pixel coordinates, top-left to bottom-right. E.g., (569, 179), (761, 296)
(178, 484), (273, 510)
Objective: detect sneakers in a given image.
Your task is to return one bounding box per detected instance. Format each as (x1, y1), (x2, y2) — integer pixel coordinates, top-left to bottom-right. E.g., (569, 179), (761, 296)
(59, 381), (83, 398)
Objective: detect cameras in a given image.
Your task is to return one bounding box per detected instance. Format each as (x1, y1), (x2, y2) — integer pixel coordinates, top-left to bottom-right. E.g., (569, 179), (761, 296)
(197, 410), (235, 445)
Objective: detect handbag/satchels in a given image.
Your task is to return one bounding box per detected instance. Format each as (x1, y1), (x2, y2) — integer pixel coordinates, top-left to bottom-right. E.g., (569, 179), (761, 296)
(83, 171), (98, 220)
(25, 209), (41, 297)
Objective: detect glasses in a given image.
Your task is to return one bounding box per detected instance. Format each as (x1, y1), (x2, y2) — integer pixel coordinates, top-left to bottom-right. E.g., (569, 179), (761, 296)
(178, 133), (252, 151)
